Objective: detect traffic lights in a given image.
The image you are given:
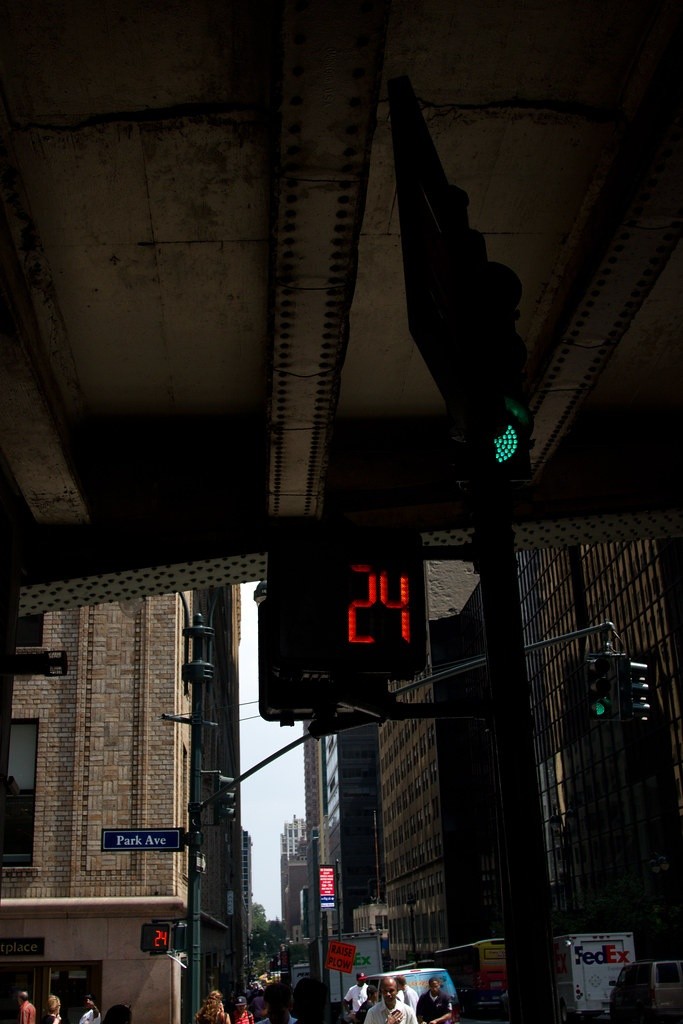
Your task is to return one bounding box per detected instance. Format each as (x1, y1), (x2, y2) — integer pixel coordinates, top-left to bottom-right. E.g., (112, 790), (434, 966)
(267, 517), (430, 680)
(584, 653), (621, 722)
(617, 651), (651, 723)
(140, 922), (172, 954)
(459, 257), (536, 484)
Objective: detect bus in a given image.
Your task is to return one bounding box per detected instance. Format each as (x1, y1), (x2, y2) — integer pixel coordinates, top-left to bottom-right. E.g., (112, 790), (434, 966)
(431, 937), (507, 1019)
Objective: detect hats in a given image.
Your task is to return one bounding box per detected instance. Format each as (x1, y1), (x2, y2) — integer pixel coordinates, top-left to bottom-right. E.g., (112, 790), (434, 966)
(235, 996), (247, 1005)
(367, 985), (380, 993)
(356, 973), (367, 979)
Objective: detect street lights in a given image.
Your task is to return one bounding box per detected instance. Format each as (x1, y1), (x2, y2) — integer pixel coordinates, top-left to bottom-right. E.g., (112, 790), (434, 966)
(176, 580), (267, 1024)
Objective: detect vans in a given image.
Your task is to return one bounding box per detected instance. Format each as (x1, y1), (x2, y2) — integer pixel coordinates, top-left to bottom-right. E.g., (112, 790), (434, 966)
(365, 968), (462, 1024)
(608, 959), (683, 1024)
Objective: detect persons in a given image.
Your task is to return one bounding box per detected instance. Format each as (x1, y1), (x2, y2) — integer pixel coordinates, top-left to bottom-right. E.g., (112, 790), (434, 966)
(42, 995), (63, 1024)
(194, 978), (328, 1024)
(342, 972), (452, 1024)
(78, 993), (102, 1024)
(101, 1004), (133, 1024)
(18, 991), (36, 1024)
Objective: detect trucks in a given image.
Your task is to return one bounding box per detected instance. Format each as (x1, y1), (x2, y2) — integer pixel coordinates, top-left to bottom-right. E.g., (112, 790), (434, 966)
(552, 930), (636, 1024)
(259, 961), (311, 993)
(307, 930), (385, 1013)
